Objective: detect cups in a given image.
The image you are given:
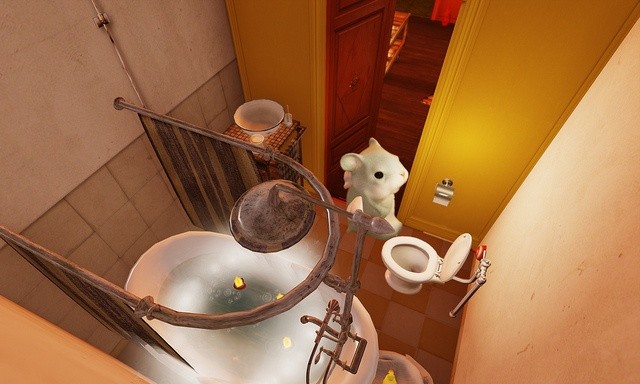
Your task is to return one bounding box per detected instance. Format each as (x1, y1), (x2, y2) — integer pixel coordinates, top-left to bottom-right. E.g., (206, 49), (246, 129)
(250, 134), (265, 154)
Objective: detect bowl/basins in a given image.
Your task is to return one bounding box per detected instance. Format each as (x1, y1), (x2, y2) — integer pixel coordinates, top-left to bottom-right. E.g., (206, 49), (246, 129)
(233, 99), (285, 136)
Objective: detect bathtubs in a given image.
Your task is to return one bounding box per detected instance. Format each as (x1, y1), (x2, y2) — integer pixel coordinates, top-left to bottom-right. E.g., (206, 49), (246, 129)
(124, 229), (379, 384)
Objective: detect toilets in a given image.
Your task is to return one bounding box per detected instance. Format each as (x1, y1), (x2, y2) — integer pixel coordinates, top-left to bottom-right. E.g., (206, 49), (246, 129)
(380, 232), (475, 297)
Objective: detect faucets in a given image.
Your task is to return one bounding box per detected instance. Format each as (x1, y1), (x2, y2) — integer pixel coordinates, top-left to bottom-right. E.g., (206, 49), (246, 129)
(300, 315), (341, 341)
